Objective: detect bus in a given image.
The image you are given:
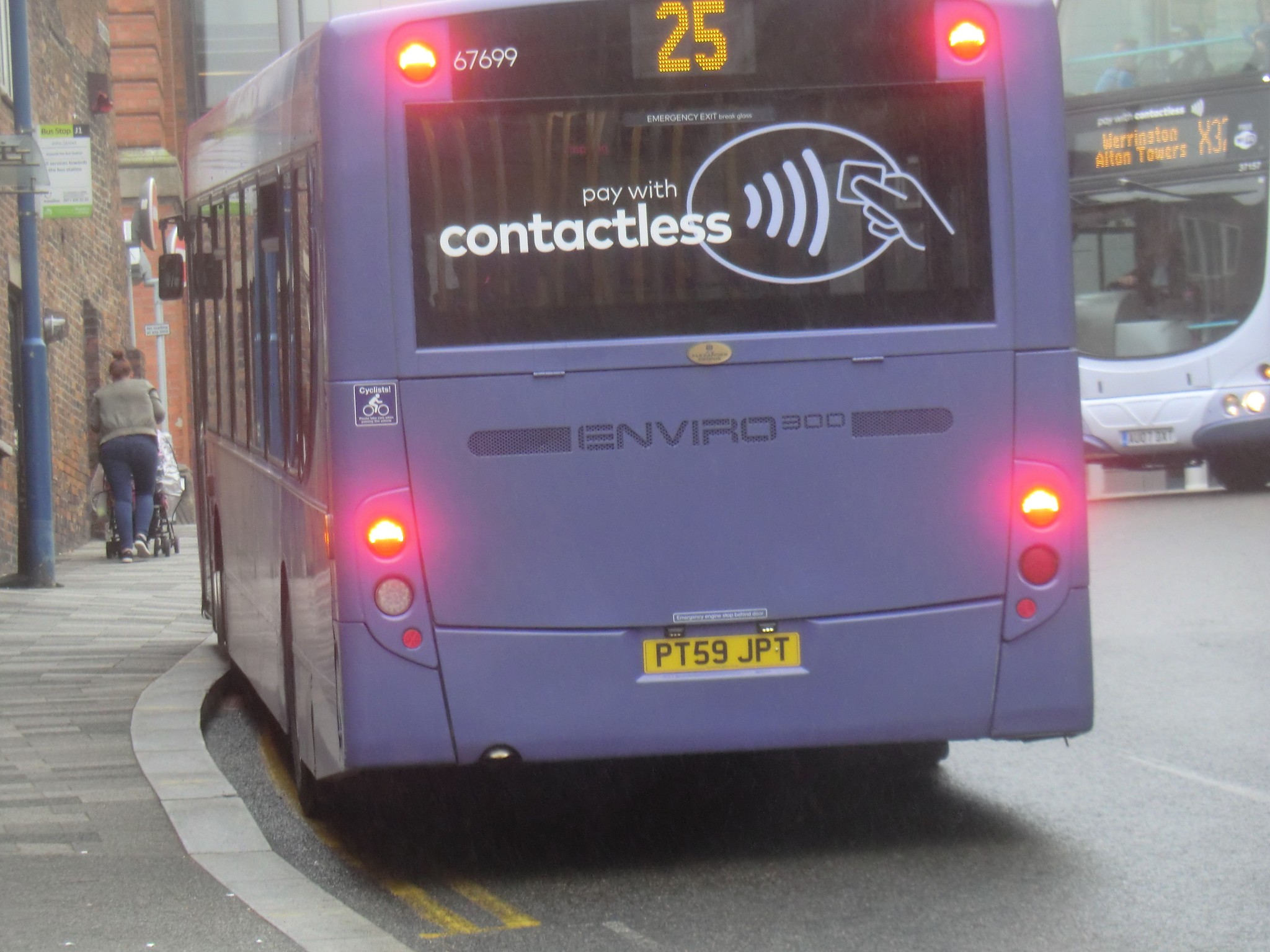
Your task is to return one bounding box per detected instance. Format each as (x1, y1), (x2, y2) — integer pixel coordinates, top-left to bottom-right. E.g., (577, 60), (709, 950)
(1050, 70), (1269, 479)
(153, 0), (1100, 803)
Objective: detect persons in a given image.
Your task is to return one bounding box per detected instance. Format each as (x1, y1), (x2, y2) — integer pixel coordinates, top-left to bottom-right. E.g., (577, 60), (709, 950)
(91, 349), (166, 564)
(1112, 228), (1196, 314)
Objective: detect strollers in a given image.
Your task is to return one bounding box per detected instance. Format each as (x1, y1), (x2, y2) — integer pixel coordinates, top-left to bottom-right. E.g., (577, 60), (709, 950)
(95, 434), (189, 554)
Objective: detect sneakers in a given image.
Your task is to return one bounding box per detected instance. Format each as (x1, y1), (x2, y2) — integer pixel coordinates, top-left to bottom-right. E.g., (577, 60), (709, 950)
(119, 550), (133, 563)
(134, 536), (151, 557)
